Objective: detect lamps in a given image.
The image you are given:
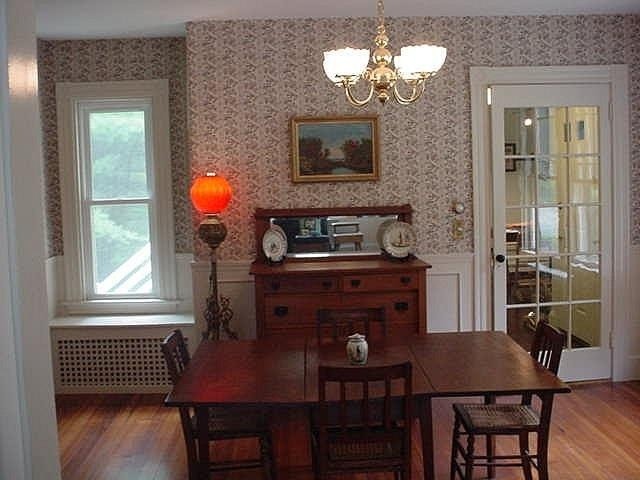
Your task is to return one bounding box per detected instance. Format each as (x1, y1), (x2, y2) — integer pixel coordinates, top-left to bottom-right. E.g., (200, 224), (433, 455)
(189, 170), (239, 341)
(322, 0), (448, 107)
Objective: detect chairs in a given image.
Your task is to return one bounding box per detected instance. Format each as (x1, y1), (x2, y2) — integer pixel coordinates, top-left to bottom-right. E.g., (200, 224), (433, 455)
(450, 319), (565, 479)
(310, 360), (412, 480)
(316, 307), (387, 343)
(160, 328), (276, 480)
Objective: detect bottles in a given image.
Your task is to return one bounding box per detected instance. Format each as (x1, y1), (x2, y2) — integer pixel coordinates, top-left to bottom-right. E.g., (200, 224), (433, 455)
(346, 333), (368, 366)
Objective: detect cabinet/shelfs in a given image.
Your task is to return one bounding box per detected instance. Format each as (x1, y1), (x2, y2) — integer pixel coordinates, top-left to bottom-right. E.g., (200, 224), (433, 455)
(260, 256), (432, 337)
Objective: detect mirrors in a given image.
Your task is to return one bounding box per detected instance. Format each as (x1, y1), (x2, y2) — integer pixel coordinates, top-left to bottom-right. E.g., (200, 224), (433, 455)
(255, 204), (413, 256)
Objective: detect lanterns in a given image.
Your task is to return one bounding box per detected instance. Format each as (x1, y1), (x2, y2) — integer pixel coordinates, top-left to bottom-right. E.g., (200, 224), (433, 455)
(191, 175), (232, 213)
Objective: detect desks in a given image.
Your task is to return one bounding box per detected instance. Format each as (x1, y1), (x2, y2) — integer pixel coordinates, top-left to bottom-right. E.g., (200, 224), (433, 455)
(164, 331), (572, 480)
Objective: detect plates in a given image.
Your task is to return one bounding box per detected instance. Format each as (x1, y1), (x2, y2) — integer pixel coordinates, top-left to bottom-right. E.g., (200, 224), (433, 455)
(262, 228), (285, 262)
(382, 222), (417, 258)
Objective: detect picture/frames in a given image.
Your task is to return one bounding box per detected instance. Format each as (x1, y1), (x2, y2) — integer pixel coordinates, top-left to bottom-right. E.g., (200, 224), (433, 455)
(290, 114), (380, 183)
(505, 143), (517, 172)
